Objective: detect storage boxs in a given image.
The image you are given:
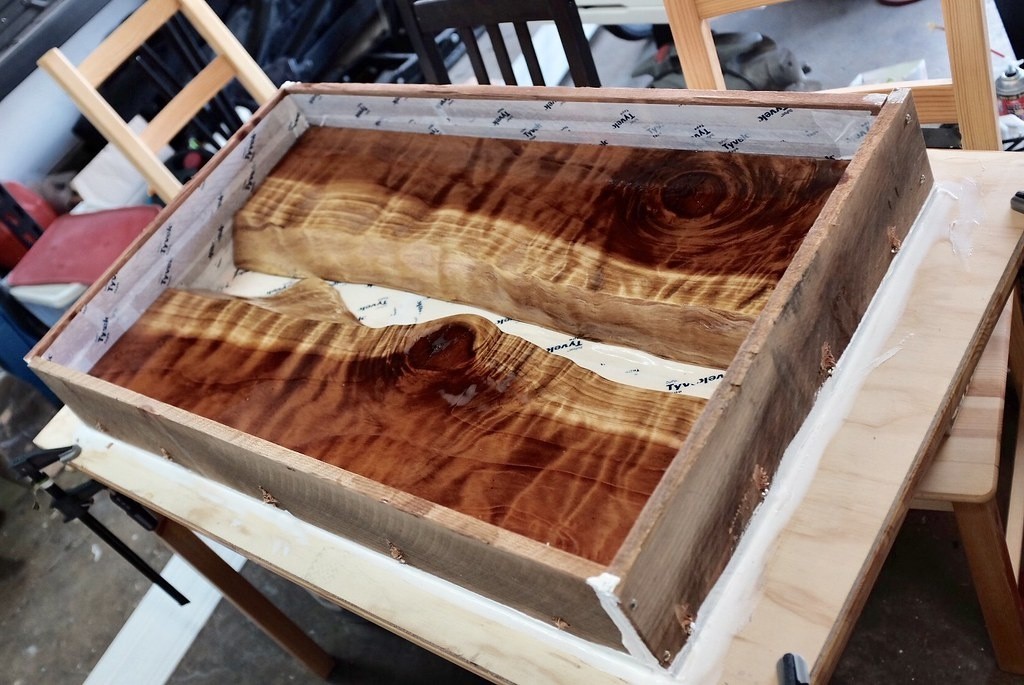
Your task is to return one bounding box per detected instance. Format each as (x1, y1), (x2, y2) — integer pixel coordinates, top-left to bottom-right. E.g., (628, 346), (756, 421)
(20, 81), (932, 671)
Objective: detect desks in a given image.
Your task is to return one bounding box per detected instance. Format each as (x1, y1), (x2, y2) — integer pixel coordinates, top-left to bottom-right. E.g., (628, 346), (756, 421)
(29, 141), (1024, 685)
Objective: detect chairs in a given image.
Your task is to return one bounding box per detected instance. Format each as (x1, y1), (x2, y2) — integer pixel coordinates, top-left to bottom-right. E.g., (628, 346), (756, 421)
(657, 0), (1024, 675)
(0, 0), (598, 331)
